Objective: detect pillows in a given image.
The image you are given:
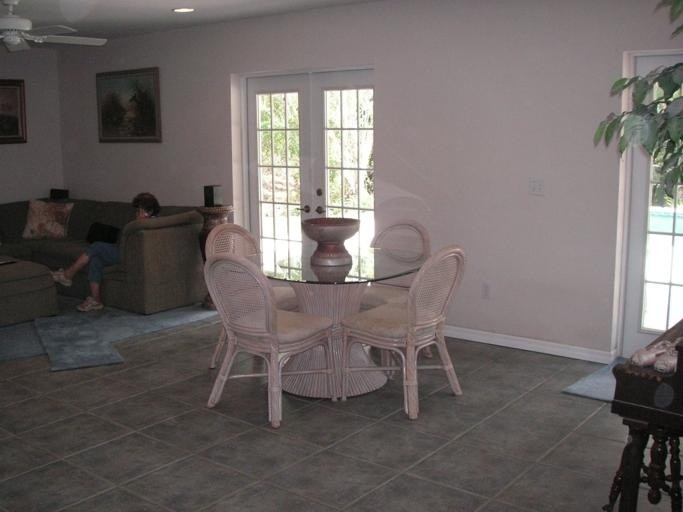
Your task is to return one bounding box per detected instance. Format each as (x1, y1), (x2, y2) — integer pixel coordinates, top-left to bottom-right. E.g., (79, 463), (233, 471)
(21, 198), (74, 242)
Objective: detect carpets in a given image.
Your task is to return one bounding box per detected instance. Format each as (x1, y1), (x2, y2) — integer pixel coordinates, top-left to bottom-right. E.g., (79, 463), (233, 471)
(560, 356), (628, 404)
(0, 292), (218, 373)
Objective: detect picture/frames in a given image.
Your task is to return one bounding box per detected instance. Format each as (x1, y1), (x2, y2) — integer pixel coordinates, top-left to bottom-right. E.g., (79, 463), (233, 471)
(95, 66), (162, 143)
(0, 78), (27, 144)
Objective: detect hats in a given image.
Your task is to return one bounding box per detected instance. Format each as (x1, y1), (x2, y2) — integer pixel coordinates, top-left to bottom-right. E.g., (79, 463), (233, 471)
(131, 193), (160, 216)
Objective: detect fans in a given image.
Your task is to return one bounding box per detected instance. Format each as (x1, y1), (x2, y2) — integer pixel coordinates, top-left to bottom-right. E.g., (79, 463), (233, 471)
(0, 0), (108, 52)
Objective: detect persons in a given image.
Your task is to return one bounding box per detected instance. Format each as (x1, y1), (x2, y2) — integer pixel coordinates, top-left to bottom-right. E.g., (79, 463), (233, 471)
(46, 191), (161, 313)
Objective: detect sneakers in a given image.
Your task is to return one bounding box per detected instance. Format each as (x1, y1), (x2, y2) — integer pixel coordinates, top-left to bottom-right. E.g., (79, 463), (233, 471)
(76, 296), (104, 313)
(48, 268), (73, 288)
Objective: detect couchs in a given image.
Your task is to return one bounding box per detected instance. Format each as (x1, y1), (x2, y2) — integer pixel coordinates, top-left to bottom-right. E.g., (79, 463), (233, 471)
(0, 197), (209, 315)
